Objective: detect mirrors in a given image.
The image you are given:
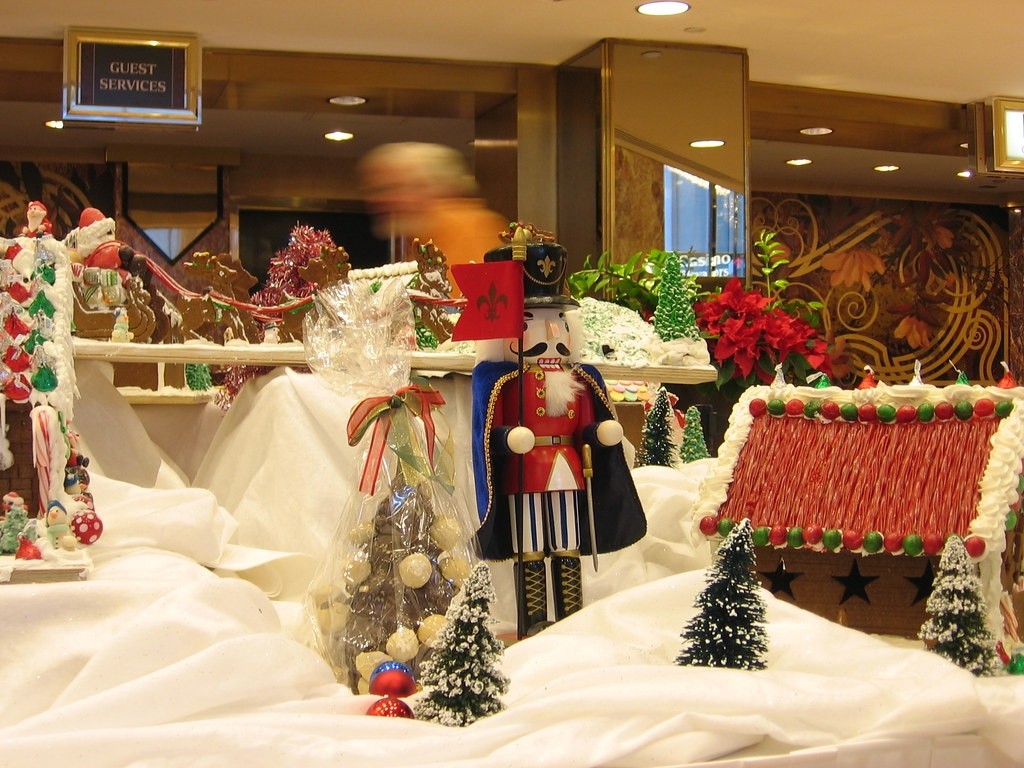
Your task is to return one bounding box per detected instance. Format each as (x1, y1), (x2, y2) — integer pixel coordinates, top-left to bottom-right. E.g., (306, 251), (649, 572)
(121, 161), (224, 264)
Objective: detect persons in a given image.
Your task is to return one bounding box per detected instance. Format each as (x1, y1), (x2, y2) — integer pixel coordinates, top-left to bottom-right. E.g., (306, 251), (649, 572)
(77, 207), (132, 286)
(17, 201), (52, 238)
(462, 228), (649, 639)
(361, 142), (508, 271)
